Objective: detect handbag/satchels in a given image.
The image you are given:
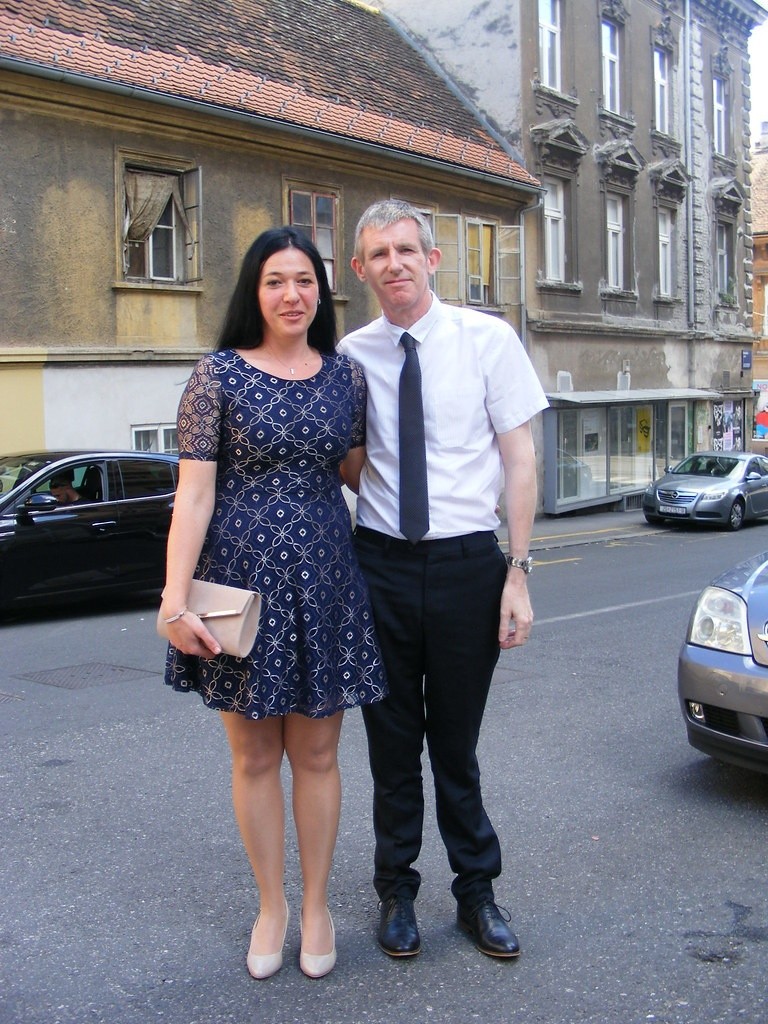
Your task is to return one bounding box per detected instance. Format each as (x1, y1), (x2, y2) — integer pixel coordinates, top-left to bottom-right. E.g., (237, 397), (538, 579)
(156, 578), (262, 658)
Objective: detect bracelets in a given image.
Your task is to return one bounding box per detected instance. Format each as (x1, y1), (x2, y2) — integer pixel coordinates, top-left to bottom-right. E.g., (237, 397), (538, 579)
(160, 604), (188, 624)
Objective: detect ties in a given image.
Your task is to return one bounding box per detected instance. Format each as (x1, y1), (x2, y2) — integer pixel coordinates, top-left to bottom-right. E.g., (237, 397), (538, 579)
(396, 332), (431, 546)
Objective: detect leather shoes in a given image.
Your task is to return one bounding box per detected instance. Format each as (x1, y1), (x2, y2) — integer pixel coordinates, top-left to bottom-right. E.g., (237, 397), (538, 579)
(456, 899), (521, 957)
(376, 897), (421, 957)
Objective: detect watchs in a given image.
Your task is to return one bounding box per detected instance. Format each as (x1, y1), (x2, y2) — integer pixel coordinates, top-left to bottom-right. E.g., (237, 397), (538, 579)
(506, 555), (533, 573)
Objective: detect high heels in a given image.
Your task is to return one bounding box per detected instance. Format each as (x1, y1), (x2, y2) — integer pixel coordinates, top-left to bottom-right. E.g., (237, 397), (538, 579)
(245, 896), (290, 979)
(298, 904), (338, 980)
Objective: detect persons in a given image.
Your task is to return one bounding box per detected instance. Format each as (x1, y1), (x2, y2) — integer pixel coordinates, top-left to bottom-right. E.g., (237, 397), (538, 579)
(49, 470), (90, 505)
(156, 226), (367, 978)
(336, 200), (550, 957)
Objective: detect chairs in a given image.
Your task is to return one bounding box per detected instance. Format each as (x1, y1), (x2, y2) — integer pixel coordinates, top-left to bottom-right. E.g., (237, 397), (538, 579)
(85, 465), (171, 502)
(700, 460), (767, 475)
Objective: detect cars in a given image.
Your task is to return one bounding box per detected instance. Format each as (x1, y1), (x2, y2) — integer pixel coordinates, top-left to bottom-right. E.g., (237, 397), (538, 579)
(641, 450), (768, 531)
(676, 549), (767, 775)
(1, 448), (180, 625)
